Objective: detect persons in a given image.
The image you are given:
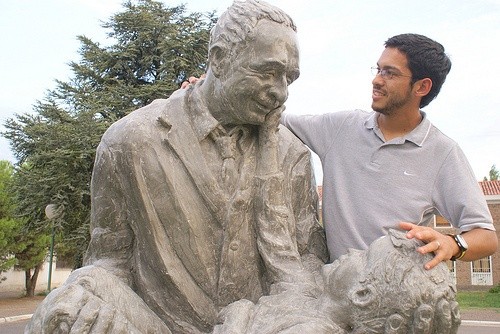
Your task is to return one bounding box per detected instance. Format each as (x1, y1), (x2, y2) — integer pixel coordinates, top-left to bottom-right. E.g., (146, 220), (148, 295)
(78, 0), (335, 334)
(25, 229), (465, 334)
(180, 32), (497, 273)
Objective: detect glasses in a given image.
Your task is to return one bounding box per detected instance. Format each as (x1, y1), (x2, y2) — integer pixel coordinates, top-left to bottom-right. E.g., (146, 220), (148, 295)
(370, 65), (425, 80)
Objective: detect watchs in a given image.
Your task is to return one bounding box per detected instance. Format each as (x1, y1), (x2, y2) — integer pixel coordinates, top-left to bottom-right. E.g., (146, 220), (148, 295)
(446, 232), (469, 261)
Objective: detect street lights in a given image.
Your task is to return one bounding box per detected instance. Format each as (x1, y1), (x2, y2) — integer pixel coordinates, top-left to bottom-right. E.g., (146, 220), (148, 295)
(44, 200), (66, 295)
